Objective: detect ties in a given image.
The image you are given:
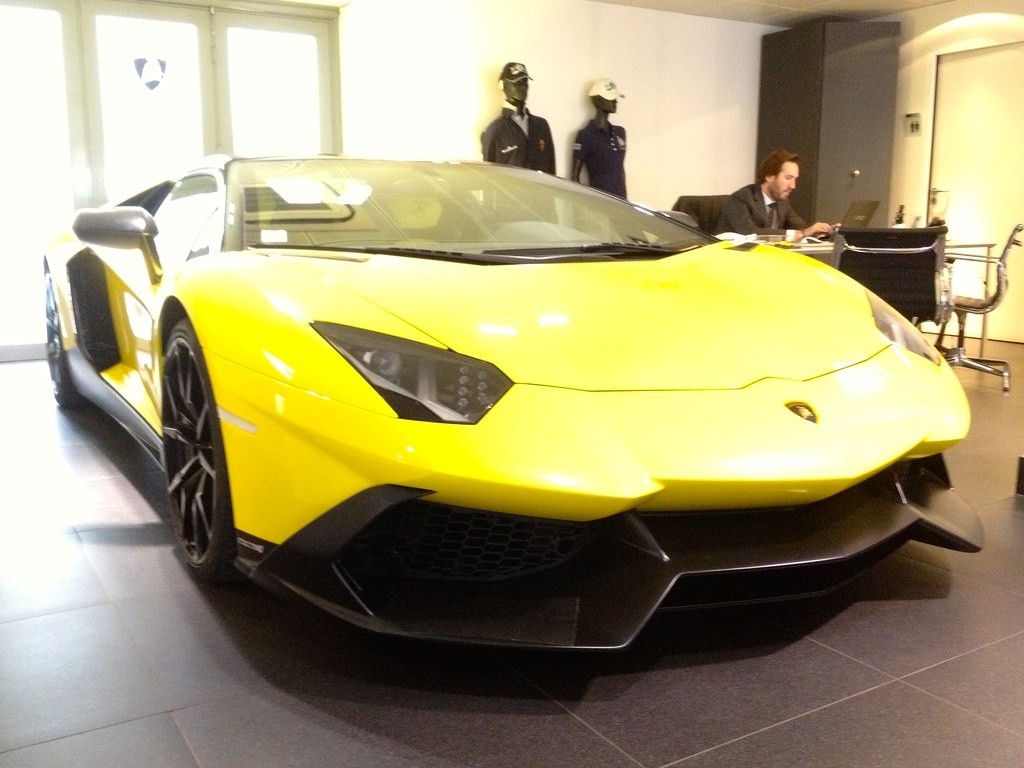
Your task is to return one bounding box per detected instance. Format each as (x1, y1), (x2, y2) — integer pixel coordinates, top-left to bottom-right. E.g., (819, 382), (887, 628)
(768, 204), (781, 229)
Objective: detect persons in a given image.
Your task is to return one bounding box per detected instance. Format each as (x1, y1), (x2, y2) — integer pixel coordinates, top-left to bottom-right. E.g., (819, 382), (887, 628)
(480, 62), (555, 175)
(716, 148), (841, 245)
(572, 79), (627, 200)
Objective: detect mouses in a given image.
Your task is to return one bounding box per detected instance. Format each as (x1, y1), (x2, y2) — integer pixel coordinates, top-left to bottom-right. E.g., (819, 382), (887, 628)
(800, 236), (822, 244)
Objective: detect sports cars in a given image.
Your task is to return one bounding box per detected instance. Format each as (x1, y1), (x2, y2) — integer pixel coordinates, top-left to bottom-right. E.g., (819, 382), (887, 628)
(38, 154), (986, 650)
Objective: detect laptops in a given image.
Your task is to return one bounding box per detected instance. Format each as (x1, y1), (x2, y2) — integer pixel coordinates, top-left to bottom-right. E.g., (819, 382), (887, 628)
(818, 201), (881, 239)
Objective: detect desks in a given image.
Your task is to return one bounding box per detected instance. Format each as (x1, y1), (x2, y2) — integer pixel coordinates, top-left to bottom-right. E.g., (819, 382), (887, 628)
(754, 241), (833, 267)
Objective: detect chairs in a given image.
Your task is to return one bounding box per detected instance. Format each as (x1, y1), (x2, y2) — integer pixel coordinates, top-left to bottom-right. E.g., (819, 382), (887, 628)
(832, 226), (954, 326)
(671, 195), (730, 235)
(934, 224), (1024, 392)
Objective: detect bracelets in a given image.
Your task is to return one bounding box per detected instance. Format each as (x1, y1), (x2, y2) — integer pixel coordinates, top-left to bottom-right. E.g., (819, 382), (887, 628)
(802, 228), (805, 239)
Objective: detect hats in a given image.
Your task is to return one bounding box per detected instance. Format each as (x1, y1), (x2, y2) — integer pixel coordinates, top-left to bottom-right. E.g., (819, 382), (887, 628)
(588, 80), (624, 100)
(500, 62), (533, 82)
